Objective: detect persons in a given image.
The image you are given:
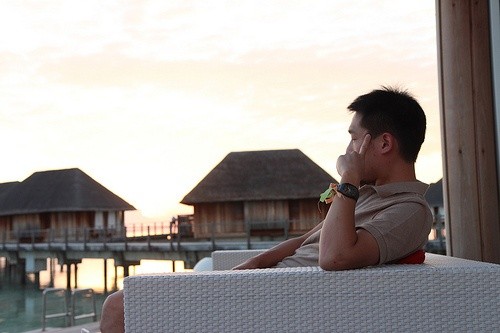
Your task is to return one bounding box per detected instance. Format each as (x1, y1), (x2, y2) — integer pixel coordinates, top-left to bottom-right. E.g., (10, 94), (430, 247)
(99, 84), (437, 333)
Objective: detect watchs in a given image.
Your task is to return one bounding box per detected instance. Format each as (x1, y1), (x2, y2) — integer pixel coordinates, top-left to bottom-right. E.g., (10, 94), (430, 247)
(334, 180), (360, 202)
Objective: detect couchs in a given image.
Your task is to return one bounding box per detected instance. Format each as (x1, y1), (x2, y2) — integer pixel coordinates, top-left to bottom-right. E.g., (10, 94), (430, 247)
(123, 249), (500, 333)
